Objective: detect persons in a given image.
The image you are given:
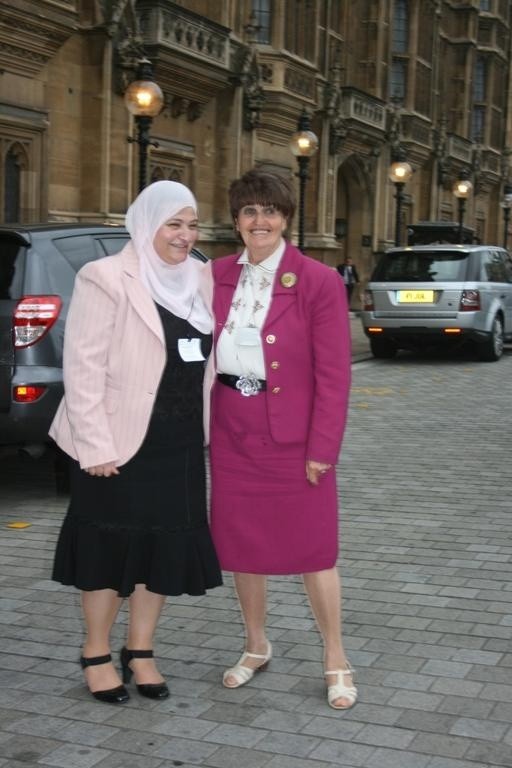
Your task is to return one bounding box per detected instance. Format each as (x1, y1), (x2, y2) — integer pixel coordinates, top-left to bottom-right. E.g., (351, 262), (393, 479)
(47, 179), (216, 705)
(202, 168), (358, 710)
(336, 257), (360, 310)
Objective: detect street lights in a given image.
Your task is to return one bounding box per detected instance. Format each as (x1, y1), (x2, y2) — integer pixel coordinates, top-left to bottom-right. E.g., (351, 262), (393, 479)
(498, 184), (512, 248)
(287, 106), (320, 254)
(123, 54), (164, 197)
(452, 170), (473, 243)
(387, 143), (412, 247)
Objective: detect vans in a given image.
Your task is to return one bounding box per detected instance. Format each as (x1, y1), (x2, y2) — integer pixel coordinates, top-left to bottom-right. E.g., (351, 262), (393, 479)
(0, 222), (215, 459)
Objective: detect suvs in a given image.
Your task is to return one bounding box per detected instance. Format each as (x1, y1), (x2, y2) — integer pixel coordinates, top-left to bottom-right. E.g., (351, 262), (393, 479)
(357, 237), (512, 360)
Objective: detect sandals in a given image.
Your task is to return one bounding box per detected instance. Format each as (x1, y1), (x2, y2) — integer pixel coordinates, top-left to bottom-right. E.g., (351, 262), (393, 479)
(223, 638), (271, 689)
(324, 659), (357, 710)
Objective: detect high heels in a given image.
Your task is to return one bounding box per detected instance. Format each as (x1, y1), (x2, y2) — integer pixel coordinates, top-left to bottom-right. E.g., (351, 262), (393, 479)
(120, 646), (169, 699)
(81, 654), (129, 702)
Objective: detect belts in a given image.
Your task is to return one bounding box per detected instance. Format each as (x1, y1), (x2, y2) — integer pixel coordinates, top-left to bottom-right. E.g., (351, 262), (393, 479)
(218, 373), (266, 396)
